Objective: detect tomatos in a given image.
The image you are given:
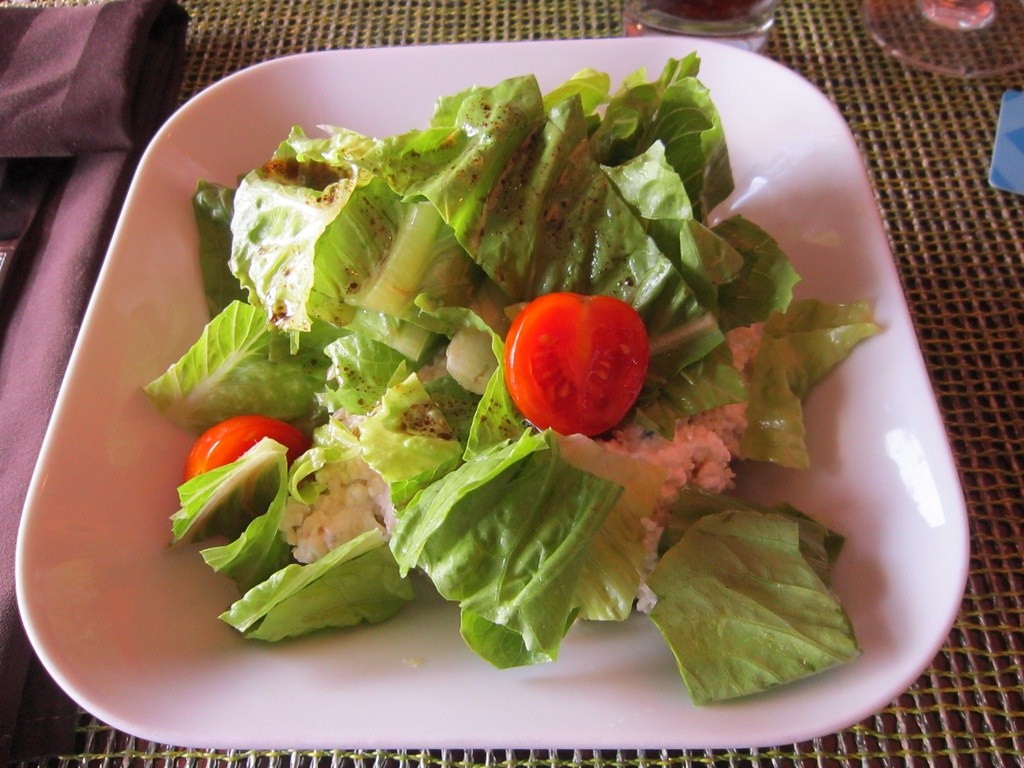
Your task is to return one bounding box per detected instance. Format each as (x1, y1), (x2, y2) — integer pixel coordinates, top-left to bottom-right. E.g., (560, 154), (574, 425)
(182, 413), (309, 486)
(502, 292), (650, 438)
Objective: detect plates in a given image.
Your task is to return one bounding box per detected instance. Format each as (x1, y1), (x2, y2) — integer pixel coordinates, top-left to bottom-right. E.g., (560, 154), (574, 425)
(14, 33), (969, 748)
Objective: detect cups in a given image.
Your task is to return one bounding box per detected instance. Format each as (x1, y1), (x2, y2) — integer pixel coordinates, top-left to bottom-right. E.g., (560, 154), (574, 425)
(619, 0), (776, 54)
(861, 0), (1024, 78)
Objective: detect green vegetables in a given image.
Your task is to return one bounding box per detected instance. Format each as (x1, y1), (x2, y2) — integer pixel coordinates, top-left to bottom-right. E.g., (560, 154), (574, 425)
(134, 49), (879, 704)
